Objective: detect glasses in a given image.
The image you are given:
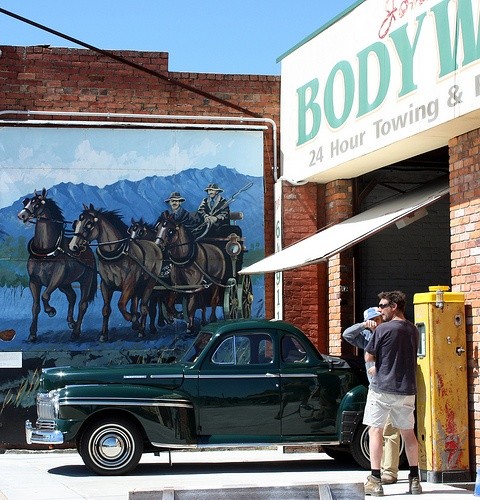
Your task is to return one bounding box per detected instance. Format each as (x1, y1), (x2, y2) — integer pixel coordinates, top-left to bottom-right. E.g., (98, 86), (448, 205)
(379, 303), (392, 308)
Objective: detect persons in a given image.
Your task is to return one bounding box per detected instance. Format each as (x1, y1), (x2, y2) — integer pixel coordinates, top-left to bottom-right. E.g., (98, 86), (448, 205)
(343, 307), (401, 485)
(362, 291), (422, 497)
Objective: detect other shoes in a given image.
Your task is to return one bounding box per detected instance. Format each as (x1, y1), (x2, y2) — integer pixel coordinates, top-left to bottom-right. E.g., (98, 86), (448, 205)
(381, 474), (398, 483)
(408, 474), (422, 494)
(364, 475), (384, 497)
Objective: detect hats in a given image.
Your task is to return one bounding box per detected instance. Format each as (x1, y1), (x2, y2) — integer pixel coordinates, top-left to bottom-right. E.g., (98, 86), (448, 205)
(364, 307), (382, 321)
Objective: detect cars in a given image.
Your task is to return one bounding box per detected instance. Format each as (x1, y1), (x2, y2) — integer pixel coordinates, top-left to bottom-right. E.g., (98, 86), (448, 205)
(25, 319), (418, 475)
